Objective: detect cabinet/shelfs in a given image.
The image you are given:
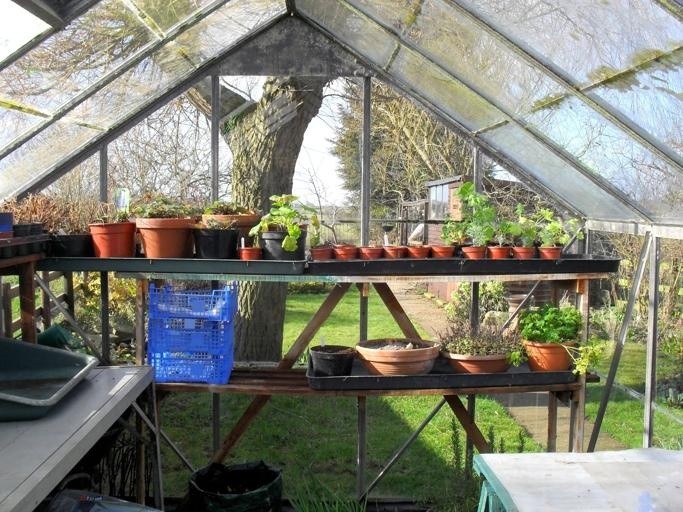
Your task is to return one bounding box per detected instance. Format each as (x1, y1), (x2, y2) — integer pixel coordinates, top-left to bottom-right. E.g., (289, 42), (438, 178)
(0, 254), (607, 512)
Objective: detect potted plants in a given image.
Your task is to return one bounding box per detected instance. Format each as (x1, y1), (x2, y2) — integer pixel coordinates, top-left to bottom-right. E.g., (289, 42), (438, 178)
(439, 302), (610, 378)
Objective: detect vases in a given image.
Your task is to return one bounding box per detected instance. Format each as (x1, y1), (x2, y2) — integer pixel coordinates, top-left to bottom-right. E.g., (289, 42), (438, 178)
(309, 336), (440, 376)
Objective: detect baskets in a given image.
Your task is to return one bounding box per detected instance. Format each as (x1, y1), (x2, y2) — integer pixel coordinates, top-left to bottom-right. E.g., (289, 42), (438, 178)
(146, 282), (238, 384)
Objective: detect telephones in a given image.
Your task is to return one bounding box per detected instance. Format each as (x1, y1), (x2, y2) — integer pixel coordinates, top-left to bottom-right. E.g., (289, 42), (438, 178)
(188, 459), (284, 512)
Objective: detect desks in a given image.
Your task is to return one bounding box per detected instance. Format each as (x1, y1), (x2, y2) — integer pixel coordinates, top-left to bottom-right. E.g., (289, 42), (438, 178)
(471, 446), (681, 512)
